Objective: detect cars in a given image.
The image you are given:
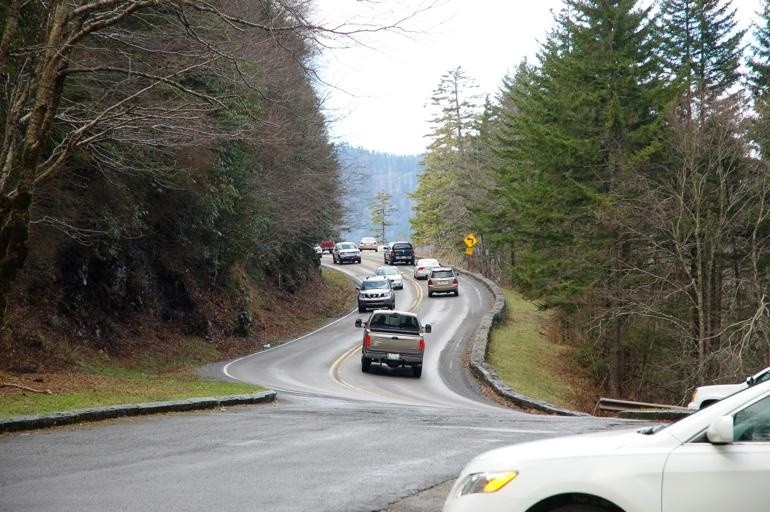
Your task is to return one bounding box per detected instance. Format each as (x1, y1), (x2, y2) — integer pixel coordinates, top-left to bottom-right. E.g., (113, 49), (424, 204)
(358, 236), (378, 252)
(685, 367), (770, 413)
(376, 266), (406, 290)
(312, 242), (322, 258)
(413, 258), (442, 280)
(441, 379), (770, 512)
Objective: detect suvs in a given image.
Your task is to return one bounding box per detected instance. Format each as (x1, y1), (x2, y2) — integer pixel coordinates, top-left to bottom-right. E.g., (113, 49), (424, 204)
(332, 241), (362, 264)
(355, 274), (396, 312)
(320, 236), (335, 255)
(383, 241), (416, 266)
(426, 267), (459, 298)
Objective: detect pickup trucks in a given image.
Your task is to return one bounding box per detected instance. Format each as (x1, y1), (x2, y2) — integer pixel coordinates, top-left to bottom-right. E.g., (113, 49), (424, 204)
(354, 308), (433, 378)
